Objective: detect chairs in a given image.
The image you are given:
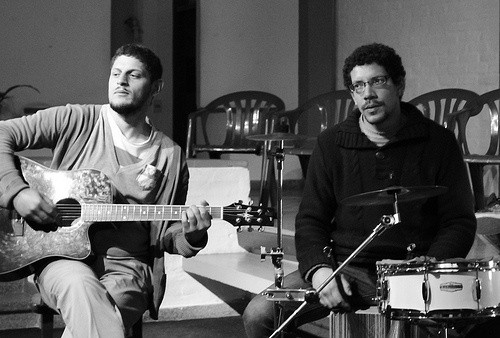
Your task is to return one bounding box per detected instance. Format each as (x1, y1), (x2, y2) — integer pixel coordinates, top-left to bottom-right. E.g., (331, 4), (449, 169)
(183, 88), (500, 223)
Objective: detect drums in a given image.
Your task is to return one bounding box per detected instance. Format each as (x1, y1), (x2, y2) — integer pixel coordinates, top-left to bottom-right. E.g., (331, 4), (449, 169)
(424, 258), (500, 318)
(376, 263), (439, 326)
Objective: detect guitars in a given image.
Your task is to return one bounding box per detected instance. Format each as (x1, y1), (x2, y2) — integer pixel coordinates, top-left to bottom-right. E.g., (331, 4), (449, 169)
(0, 152), (274, 282)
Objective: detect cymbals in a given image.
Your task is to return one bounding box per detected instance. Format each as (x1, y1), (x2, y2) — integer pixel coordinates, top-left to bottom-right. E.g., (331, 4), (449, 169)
(339, 185), (448, 207)
(246, 134), (305, 141)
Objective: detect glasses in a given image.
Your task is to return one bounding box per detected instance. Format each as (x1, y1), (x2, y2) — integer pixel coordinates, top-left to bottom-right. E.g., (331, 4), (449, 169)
(349, 75), (390, 94)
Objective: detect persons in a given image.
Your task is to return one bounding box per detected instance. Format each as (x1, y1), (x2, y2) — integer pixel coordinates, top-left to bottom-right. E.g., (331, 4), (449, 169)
(242, 43), (478, 338)
(0, 45), (211, 338)
